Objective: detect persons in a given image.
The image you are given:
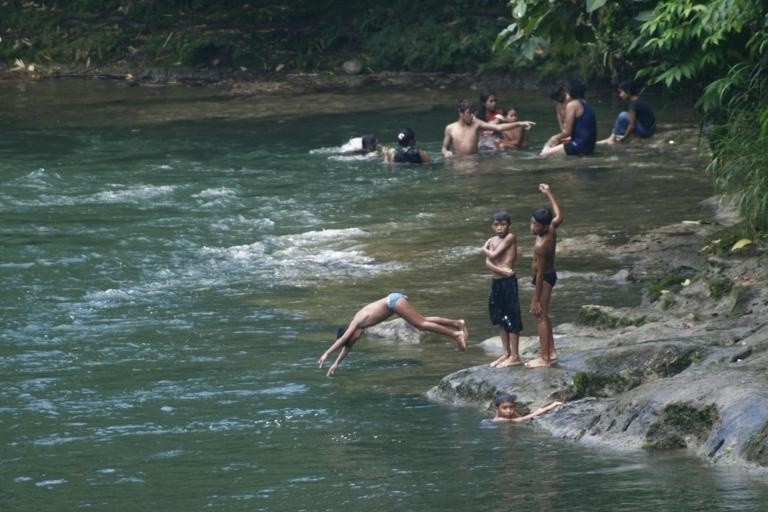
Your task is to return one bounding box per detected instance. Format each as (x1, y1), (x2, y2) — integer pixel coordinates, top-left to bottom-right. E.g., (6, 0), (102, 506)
(383, 127), (432, 164)
(495, 394), (563, 425)
(596, 81), (657, 144)
(478, 89), (504, 139)
(524, 181), (563, 368)
(318, 291), (468, 377)
(537, 81), (596, 159)
(494, 106), (528, 149)
(549, 84), (572, 132)
(479, 212), (524, 367)
(362, 134), (379, 151)
(441, 98), (536, 158)
